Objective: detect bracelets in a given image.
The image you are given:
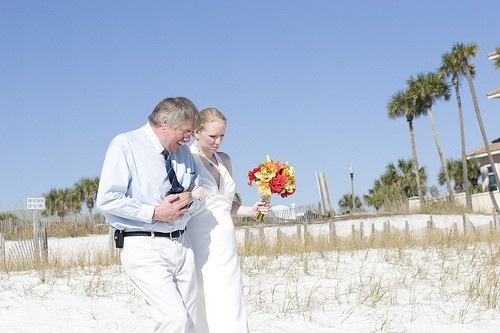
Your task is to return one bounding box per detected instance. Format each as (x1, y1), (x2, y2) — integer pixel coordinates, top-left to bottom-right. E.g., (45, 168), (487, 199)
(186, 192), (193, 202)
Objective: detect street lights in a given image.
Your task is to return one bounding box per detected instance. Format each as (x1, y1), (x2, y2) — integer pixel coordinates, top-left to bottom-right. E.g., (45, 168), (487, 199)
(349, 166), (355, 213)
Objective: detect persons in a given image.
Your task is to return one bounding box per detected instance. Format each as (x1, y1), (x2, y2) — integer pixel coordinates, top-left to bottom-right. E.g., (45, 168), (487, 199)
(187, 108), (269, 333)
(95, 96), (201, 333)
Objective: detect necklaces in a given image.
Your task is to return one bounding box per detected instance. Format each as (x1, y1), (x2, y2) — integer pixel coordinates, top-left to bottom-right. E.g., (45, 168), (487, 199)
(210, 162), (214, 168)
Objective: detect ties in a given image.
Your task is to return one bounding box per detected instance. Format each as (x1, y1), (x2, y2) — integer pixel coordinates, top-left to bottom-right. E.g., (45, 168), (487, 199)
(161, 149), (185, 197)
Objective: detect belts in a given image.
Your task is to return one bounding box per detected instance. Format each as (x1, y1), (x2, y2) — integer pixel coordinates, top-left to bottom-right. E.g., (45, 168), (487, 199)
(124, 226), (189, 241)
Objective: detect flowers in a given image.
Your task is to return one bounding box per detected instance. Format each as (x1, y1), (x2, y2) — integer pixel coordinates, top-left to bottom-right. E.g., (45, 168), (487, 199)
(247, 154), (296, 221)
